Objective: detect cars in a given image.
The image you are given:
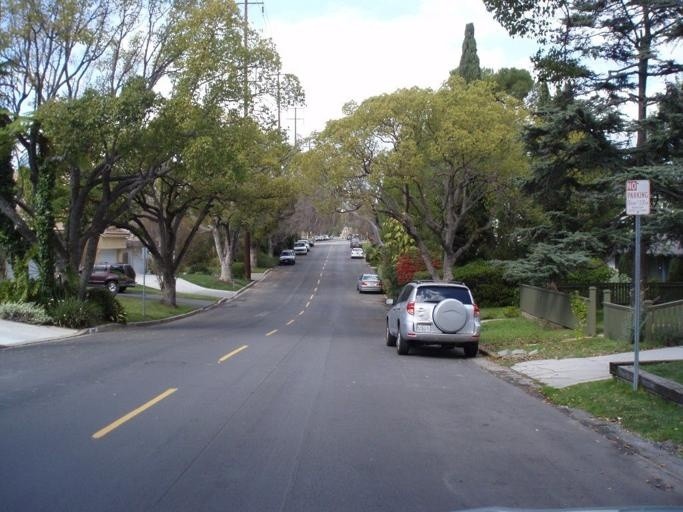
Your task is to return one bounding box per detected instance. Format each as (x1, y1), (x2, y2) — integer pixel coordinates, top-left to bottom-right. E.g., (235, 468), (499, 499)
(351, 248), (364, 258)
(350, 242), (361, 248)
(278, 249), (296, 265)
(294, 236), (335, 255)
(356, 273), (382, 293)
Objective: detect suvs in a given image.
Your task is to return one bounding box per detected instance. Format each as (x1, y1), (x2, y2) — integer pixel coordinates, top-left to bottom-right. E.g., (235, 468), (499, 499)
(384, 279), (481, 359)
(79, 263), (136, 294)
